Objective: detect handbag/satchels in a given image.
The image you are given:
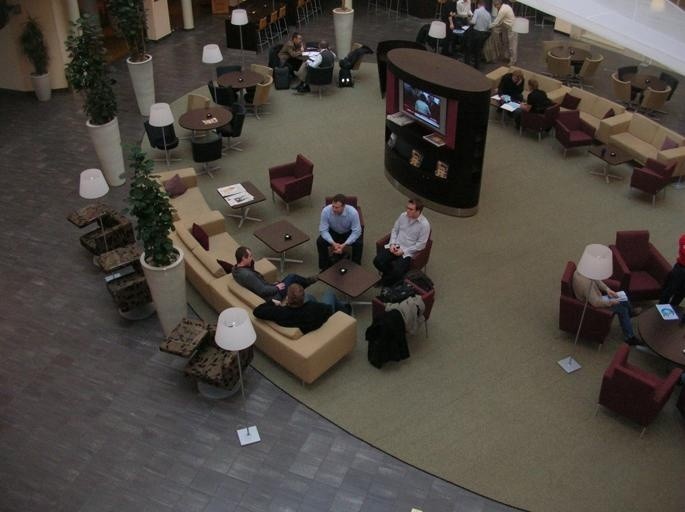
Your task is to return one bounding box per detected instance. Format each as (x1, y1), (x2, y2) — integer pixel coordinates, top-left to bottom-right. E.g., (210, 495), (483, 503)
(380, 280), (414, 303)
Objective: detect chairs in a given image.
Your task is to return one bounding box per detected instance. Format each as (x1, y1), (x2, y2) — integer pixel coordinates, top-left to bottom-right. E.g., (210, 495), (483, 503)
(618, 65), (641, 92)
(245, 75), (273, 119)
(207, 80), (239, 105)
(216, 102), (246, 153)
(143, 119), (183, 170)
(296, 0), (307, 29)
(570, 52), (604, 90)
(611, 72), (631, 101)
(191, 132), (222, 178)
(268, 44), (284, 67)
(178, 93), (210, 142)
(277, 4), (289, 40)
(641, 84), (672, 121)
(659, 71), (680, 101)
(547, 50), (571, 75)
(249, 63), (273, 104)
(388, 0), (410, 17)
(567, 40), (592, 53)
(544, 40), (564, 65)
(267, 10), (281, 44)
(256, 15), (271, 53)
(434, 0), (451, 20)
(313, 0), (323, 17)
(524, 6), (537, 26)
(305, 0), (314, 22)
(336, 42), (363, 83)
(368, 0), (388, 16)
(305, 64), (334, 100)
(217, 65), (242, 79)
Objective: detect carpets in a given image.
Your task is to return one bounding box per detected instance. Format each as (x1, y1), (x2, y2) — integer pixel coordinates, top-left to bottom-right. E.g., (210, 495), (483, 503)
(144, 64), (685, 512)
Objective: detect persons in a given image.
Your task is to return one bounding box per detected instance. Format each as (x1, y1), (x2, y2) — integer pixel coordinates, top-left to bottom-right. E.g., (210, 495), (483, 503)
(375, 272), (421, 304)
(456, 0), (473, 26)
(232, 246), (319, 304)
(291, 40), (336, 92)
(427, 94), (440, 124)
(515, 79), (553, 131)
(501, 0), (512, 62)
(277, 32), (306, 80)
(495, 70), (525, 118)
(415, 94), (432, 116)
(253, 284), (352, 335)
(572, 269), (646, 346)
(316, 194), (364, 275)
(489, 0), (518, 65)
(441, 0), (458, 58)
(374, 198), (431, 288)
(660, 234), (685, 305)
(403, 85), (421, 111)
(467, 0), (491, 70)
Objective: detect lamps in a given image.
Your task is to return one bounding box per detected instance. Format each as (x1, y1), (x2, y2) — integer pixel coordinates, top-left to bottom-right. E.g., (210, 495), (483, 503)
(428, 20), (446, 53)
(556, 244), (613, 374)
(511, 17), (530, 33)
(215, 307), (261, 447)
(149, 102), (176, 171)
(202, 43), (224, 104)
(80, 168), (121, 282)
(231, 8), (248, 71)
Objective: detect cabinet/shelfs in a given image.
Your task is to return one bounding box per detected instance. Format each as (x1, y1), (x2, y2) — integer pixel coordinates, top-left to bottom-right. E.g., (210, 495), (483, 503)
(384, 47), (493, 218)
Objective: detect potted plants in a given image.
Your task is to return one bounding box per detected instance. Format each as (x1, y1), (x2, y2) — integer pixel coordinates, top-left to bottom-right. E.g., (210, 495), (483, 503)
(12, 6), (51, 101)
(118, 139), (188, 340)
(105, 0), (156, 116)
(63, 10), (126, 186)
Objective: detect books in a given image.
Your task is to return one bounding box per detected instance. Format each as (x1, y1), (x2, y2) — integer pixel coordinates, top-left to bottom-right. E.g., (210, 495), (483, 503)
(600, 291), (627, 301)
(656, 304), (679, 320)
(387, 112), (417, 127)
(217, 183), (254, 206)
(423, 133), (446, 146)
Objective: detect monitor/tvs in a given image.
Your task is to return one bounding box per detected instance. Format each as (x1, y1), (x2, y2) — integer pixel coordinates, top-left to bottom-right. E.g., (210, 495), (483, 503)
(398, 79), (447, 136)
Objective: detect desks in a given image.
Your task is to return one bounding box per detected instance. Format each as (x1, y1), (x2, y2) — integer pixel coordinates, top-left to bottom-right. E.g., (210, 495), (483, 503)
(588, 144), (634, 184)
(178, 107), (233, 157)
(488, 98), (513, 128)
(622, 73), (667, 113)
(159, 316), (211, 359)
(551, 47), (592, 87)
(253, 219), (310, 273)
(217, 71), (265, 115)
(316, 259), (382, 312)
(96, 243), (141, 273)
(65, 201), (108, 228)
(638, 306), (684, 366)
(216, 181), (267, 228)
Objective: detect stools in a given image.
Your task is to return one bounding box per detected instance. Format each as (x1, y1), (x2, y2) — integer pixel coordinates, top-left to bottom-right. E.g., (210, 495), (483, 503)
(558, 261), (620, 351)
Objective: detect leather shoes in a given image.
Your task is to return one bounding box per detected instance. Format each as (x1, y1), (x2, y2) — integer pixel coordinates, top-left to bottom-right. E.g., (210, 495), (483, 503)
(626, 337), (644, 345)
(292, 85), (303, 89)
(629, 307), (641, 317)
(298, 87), (310, 92)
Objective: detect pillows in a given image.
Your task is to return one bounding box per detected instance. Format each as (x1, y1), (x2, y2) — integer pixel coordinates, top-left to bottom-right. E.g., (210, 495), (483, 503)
(216, 258), (234, 275)
(163, 173), (187, 199)
(560, 92), (582, 109)
(192, 222), (210, 252)
(602, 108), (616, 118)
(660, 136), (679, 151)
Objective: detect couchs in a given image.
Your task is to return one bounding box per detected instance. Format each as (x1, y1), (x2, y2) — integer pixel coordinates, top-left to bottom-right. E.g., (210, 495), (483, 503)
(485, 65), (685, 185)
(184, 323), (255, 399)
(325, 196), (365, 246)
(608, 230), (675, 303)
(519, 102), (561, 142)
(552, 110), (596, 160)
(145, 168), (357, 386)
(269, 153), (314, 215)
(105, 254), (156, 320)
(593, 342), (683, 439)
(376, 228), (434, 273)
(79, 208), (136, 267)
(372, 278), (435, 357)
(630, 157), (679, 208)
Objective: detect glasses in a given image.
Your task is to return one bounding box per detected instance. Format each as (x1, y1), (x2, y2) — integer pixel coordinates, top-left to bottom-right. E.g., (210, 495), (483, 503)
(407, 207), (418, 211)
